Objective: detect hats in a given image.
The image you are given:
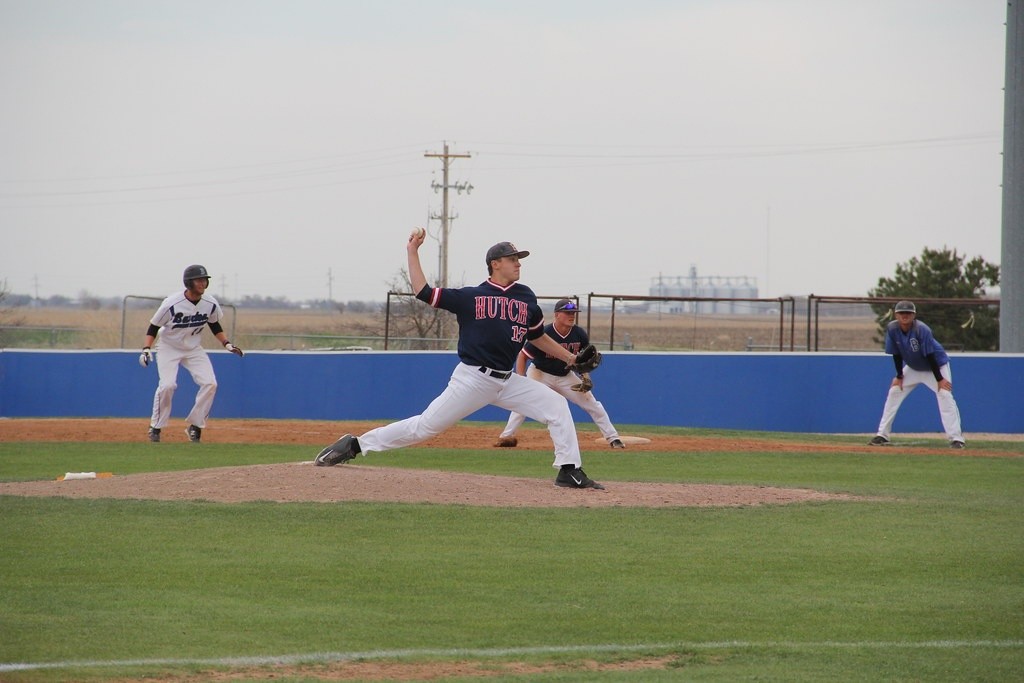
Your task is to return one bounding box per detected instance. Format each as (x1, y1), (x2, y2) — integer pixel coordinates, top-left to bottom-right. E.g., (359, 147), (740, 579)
(554, 299), (582, 312)
(486, 241), (529, 265)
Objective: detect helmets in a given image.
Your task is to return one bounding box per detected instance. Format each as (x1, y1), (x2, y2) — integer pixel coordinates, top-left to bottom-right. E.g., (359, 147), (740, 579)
(895, 301), (916, 313)
(183, 265), (211, 289)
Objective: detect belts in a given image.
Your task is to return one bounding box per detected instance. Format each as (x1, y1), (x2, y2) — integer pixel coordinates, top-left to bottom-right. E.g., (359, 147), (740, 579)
(479, 366), (512, 379)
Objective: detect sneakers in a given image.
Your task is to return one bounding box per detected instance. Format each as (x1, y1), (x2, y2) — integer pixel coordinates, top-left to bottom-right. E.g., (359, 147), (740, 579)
(148, 426), (161, 442)
(950, 440), (965, 449)
(314, 434), (356, 466)
(185, 424), (200, 442)
(611, 439), (625, 448)
(868, 436), (890, 445)
(555, 466), (605, 490)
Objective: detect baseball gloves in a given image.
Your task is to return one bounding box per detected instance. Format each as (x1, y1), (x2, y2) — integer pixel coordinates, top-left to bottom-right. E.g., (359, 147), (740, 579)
(570, 378), (592, 393)
(568, 345), (602, 373)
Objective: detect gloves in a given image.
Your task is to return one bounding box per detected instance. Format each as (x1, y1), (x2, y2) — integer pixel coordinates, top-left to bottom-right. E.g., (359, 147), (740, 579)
(224, 341), (243, 357)
(139, 347), (153, 367)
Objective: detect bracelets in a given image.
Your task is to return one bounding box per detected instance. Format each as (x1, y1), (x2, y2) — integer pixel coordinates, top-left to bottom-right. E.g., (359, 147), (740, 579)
(222, 340), (230, 347)
(567, 354), (574, 366)
(896, 375), (904, 378)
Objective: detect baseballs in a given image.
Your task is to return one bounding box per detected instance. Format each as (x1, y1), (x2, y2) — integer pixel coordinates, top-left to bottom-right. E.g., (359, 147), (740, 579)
(411, 226), (423, 238)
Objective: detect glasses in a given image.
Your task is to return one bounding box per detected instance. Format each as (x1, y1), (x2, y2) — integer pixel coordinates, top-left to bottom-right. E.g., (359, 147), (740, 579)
(556, 303), (576, 312)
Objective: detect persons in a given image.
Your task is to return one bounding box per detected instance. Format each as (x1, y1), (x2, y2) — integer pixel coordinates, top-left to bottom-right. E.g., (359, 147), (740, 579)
(313, 227), (609, 489)
(496, 299), (625, 448)
(139, 265), (245, 441)
(867, 301), (966, 451)
